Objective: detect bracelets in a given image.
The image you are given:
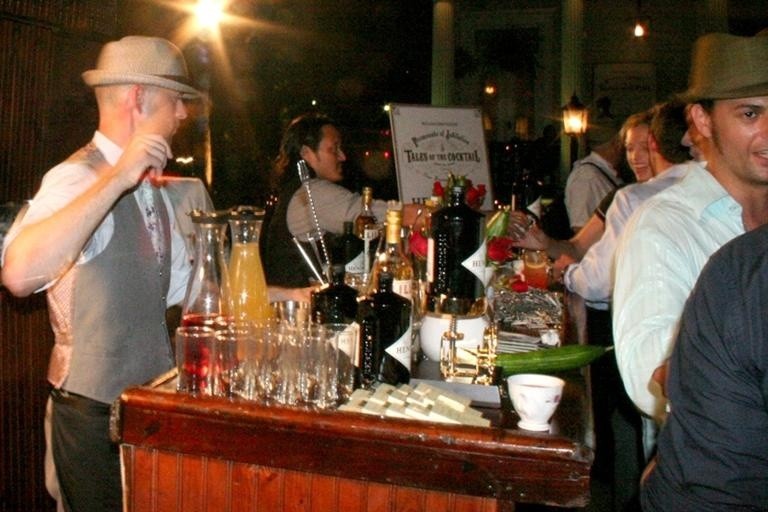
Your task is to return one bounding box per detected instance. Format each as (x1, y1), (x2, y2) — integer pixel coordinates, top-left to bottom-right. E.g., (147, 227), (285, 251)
(559, 261), (576, 285)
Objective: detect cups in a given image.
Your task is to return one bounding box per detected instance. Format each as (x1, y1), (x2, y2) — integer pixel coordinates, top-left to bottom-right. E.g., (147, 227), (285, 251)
(174, 325), (213, 399)
(229, 322), (271, 404)
(275, 320), (319, 405)
(323, 322), (358, 408)
(271, 300), (314, 328)
(507, 373), (566, 431)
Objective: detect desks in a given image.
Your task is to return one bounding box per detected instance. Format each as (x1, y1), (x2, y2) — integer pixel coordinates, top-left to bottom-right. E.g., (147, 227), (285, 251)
(109, 292), (596, 512)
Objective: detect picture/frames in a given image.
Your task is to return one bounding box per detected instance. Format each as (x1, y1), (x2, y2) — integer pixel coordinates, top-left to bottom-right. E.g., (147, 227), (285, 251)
(386, 102), (496, 213)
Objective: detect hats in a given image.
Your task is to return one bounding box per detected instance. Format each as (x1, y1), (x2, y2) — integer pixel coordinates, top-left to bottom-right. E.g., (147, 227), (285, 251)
(80, 35), (203, 99)
(674, 29), (767, 102)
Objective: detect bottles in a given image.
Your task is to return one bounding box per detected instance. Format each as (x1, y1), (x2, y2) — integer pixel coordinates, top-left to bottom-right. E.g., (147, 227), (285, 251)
(426, 184), (484, 317)
(359, 271), (412, 385)
(314, 264), (358, 321)
(355, 186), (378, 244)
(372, 208), (415, 300)
(331, 220), (364, 262)
(510, 145), (542, 251)
(225, 207), (275, 360)
(180, 207), (229, 394)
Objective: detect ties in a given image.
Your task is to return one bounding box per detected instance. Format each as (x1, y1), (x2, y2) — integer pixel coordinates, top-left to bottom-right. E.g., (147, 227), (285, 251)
(137, 183), (164, 263)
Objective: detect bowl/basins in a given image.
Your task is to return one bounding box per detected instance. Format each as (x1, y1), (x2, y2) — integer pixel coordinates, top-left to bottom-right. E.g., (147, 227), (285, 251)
(420, 316), (485, 361)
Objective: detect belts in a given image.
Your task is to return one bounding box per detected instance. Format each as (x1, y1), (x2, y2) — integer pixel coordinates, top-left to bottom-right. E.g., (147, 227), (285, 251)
(51, 389), (110, 414)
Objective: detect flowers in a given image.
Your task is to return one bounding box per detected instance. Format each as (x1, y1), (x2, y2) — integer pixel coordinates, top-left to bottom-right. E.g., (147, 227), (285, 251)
(403, 227), (429, 263)
(427, 171), (487, 209)
(485, 234), (513, 269)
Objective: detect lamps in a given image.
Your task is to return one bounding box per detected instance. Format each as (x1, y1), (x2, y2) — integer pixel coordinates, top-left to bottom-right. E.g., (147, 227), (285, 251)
(631, 16), (644, 36)
(559, 89), (589, 170)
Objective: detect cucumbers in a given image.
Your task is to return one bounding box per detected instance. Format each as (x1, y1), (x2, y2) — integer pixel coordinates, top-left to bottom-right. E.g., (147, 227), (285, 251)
(492, 343), (617, 375)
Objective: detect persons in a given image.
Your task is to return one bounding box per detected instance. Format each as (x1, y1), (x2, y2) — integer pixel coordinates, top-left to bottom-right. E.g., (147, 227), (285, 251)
(564, 120), (625, 235)
(510, 110), (656, 263)
(535, 123), (561, 169)
(258, 113), (529, 304)
(611, 30), (767, 463)
(639, 221), (767, 512)
(550, 100), (692, 311)
(156, 176), (222, 368)
(678, 92), (706, 161)
(585, 300), (644, 484)
(479, 110), (556, 215)
(0, 35), (208, 511)
